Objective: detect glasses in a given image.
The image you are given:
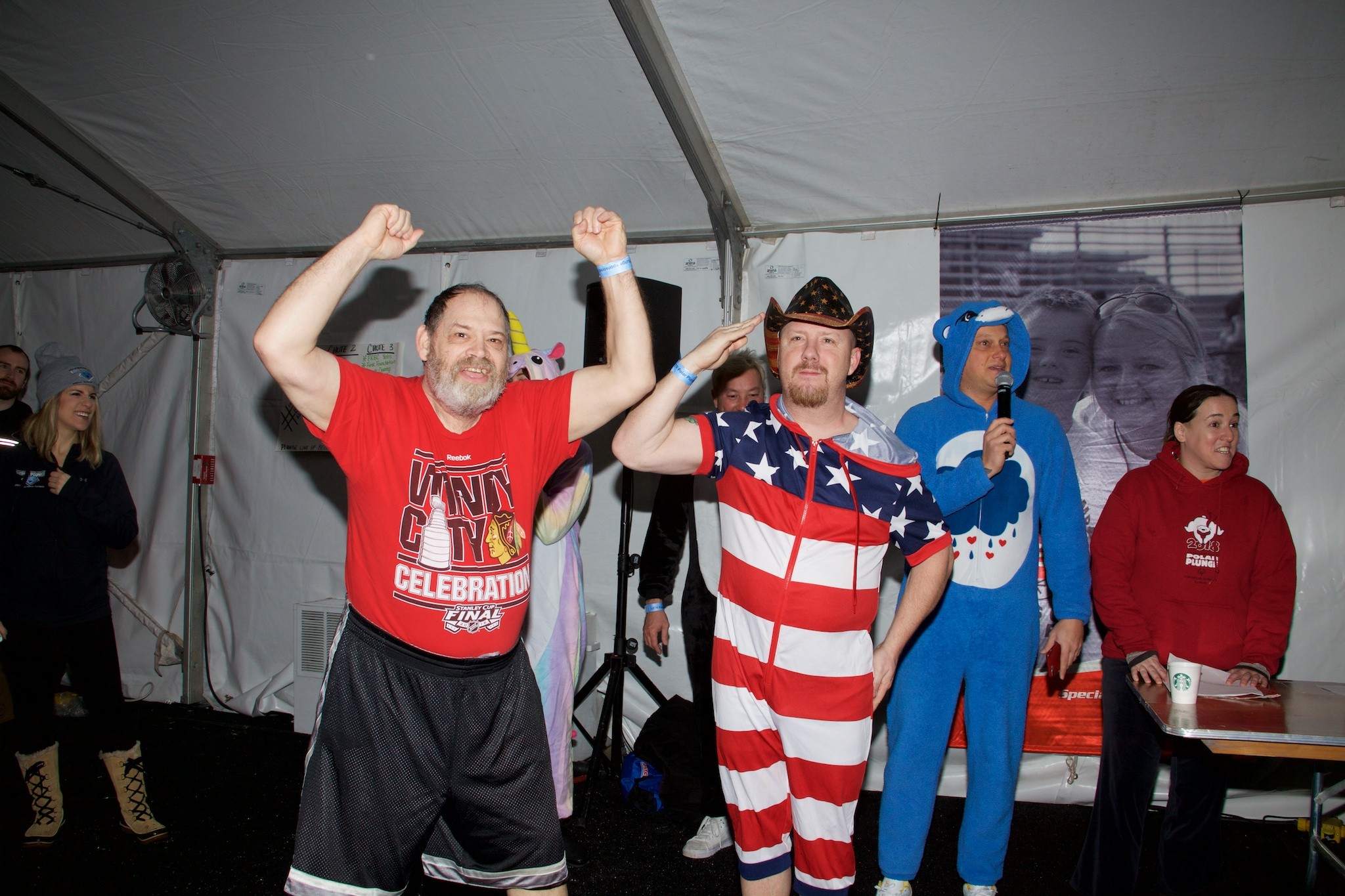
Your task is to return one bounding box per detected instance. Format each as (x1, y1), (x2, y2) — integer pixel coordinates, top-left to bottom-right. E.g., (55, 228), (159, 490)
(1093, 292), (1196, 343)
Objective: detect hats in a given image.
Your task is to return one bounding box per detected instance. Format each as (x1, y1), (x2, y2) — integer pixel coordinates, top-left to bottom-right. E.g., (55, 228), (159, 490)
(36, 356), (99, 408)
(763, 278), (875, 389)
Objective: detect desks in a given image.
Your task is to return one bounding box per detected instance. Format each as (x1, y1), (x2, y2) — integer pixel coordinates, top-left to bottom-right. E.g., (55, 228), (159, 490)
(1123, 672), (1345, 887)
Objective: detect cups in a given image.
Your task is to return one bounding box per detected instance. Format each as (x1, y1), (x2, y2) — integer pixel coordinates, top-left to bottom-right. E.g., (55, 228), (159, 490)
(1167, 662), (1202, 705)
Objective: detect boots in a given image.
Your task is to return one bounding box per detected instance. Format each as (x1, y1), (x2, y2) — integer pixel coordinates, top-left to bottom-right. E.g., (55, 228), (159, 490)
(97, 743), (169, 843)
(15, 741), (65, 848)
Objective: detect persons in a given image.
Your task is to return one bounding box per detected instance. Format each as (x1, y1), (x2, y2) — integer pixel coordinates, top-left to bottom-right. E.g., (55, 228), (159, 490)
(506, 311), (594, 819)
(1081, 383), (1296, 896)
(636, 351), (765, 855)
(1073, 284), (1249, 470)
(612, 276), (955, 896)
(871, 300), (1092, 896)
(0, 341), (168, 847)
(253, 203), (656, 896)
(1012, 285), (1127, 527)
(0, 344), (34, 451)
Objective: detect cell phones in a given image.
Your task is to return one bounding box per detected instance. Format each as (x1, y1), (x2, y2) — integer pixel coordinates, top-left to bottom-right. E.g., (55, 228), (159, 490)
(1046, 641), (1062, 677)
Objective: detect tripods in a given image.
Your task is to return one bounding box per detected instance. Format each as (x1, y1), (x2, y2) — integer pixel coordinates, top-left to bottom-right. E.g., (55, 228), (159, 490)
(572, 467), (667, 789)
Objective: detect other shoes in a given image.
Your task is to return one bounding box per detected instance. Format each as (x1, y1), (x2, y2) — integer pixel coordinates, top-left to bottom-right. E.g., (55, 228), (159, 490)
(963, 883), (996, 896)
(873, 876), (912, 896)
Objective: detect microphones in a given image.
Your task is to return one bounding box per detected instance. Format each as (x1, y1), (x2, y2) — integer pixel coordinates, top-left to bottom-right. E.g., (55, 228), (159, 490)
(994, 371), (1014, 459)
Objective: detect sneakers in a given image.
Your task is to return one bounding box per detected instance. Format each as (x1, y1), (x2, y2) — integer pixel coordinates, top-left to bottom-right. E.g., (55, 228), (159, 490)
(682, 813), (732, 859)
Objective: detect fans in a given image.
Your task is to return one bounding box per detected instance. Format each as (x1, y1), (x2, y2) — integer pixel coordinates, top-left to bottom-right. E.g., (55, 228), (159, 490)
(144, 252), (203, 332)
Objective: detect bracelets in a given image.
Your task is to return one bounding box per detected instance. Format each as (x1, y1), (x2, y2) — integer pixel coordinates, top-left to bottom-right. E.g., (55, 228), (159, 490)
(597, 256), (633, 278)
(644, 602), (664, 613)
(670, 360), (697, 386)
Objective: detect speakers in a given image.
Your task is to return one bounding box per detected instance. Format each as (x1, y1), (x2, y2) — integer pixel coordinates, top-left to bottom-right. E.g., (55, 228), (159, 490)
(584, 277), (684, 386)
